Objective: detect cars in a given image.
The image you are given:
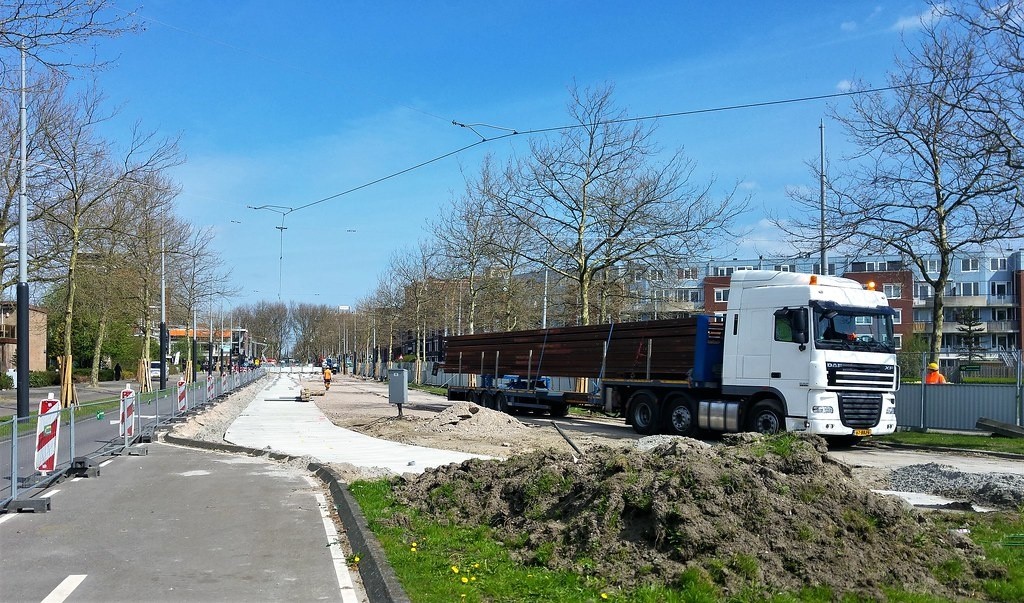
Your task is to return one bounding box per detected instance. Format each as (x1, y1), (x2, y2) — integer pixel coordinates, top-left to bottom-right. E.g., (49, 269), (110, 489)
(145, 360), (170, 381)
(201, 361), (216, 371)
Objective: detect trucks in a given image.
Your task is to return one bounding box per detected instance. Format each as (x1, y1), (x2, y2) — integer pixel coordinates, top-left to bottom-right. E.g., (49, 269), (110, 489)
(321, 357), (338, 374)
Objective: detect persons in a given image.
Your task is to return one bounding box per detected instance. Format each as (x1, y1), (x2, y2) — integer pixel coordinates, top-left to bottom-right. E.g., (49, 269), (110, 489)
(926, 363), (946, 384)
(114, 363), (122, 381)
(228, 365), (230, 369)
(776, 311), (792, 342)
(219, 366), (224, 376)
(224, 365), (226, 367)
(323, 366), (332, 391)
(232, 365), (234, 373)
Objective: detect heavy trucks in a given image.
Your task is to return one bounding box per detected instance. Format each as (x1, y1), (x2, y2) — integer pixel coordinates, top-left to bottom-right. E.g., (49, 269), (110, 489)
(438, 269), (902, 449)
